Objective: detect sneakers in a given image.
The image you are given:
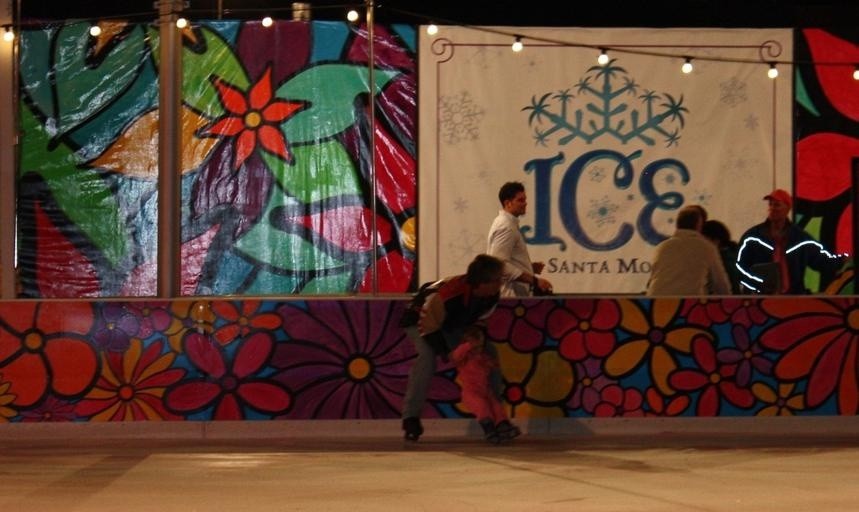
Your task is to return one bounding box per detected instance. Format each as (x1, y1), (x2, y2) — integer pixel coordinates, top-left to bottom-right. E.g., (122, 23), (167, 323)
(405, 414), (427, 442)
(491, 416), (522, 440)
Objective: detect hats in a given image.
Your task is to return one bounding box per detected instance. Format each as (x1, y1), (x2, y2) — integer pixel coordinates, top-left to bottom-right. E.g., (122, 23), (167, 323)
(761, 188), (793, 208)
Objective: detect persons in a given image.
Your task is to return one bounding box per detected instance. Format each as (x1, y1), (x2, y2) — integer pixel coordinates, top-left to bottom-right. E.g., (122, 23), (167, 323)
(702, 221), (739, 296)
(735, 189), (850, 296)
(644, 207), (733, 296)
(486, 180), (553, 298)
(401, 253), (521, 439)
